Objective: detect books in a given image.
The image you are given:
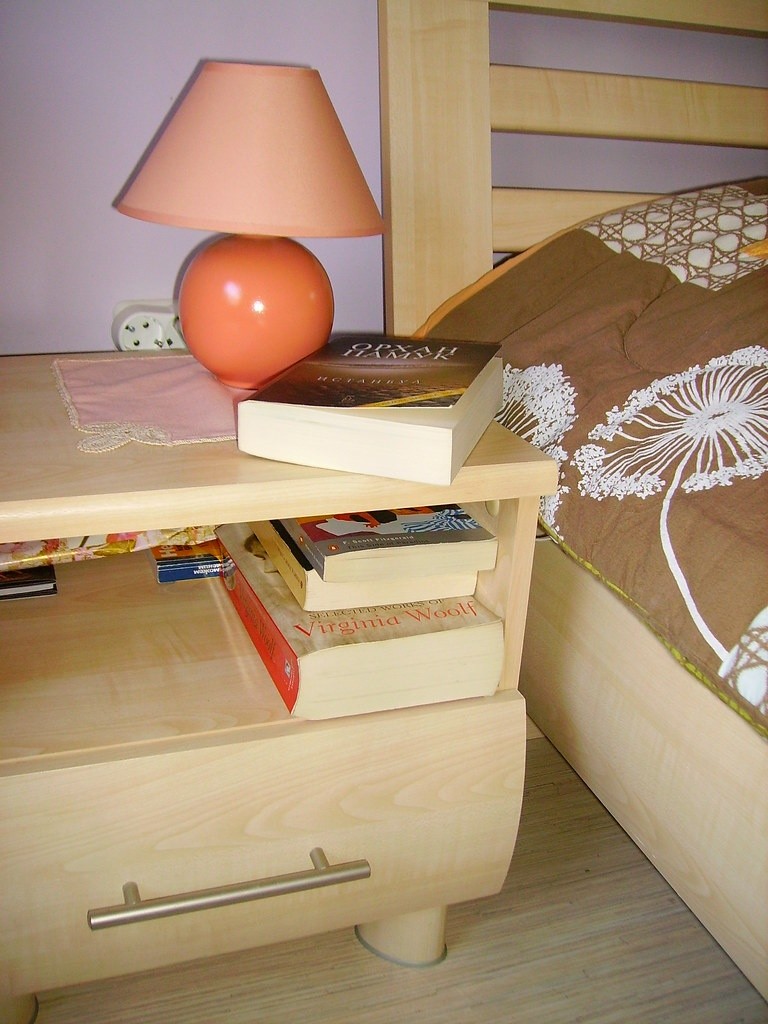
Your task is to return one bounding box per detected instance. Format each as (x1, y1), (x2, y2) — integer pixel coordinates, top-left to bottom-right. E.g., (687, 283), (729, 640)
(237, 332), (504, 486)
(0, 564), (58, 601)
(147, 503), (505, 721)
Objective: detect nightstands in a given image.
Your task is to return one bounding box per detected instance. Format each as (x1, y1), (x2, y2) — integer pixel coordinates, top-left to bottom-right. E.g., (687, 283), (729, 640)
(0, 351), (559, 1024)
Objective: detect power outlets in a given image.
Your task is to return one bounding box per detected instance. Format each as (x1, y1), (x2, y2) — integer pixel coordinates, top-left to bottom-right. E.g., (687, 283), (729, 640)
(110, 306), (189, 351)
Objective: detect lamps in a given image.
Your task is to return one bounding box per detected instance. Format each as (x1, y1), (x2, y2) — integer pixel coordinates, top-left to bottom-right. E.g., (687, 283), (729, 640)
(115, 60), (386, 390)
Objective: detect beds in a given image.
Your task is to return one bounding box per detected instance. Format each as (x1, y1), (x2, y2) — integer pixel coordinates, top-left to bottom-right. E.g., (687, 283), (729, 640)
(378, 0), (768, 1007)
